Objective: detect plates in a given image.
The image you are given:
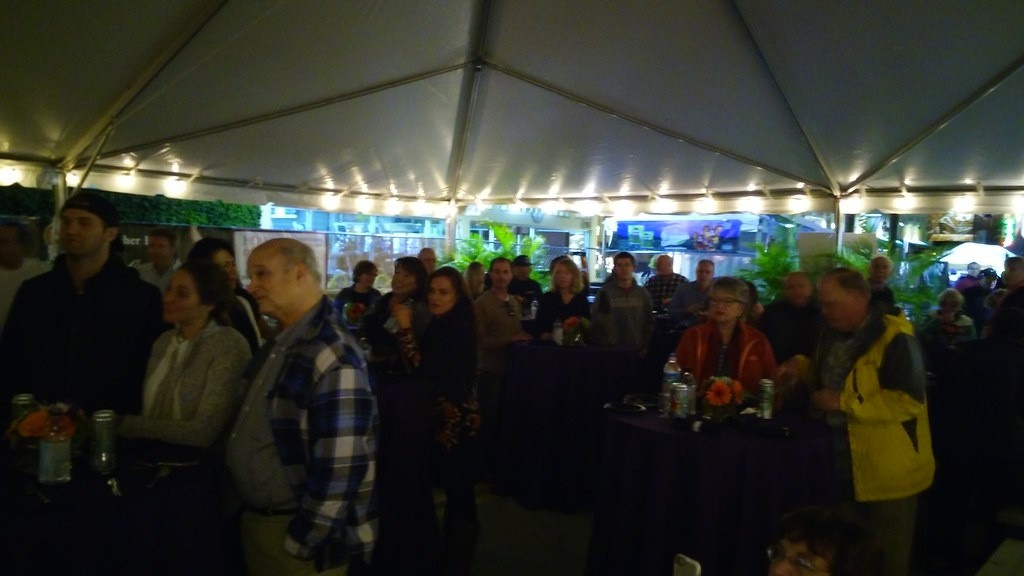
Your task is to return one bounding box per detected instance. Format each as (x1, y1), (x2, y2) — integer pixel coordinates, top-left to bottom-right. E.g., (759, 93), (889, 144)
(621, 392), (664, 408)
(605, 401), (647, 416)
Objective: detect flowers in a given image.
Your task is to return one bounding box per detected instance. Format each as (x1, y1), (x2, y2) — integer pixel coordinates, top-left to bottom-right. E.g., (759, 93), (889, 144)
(560, 315), (590, 335)
(702, 374), (745, 406)
(15, 403), (78, 441)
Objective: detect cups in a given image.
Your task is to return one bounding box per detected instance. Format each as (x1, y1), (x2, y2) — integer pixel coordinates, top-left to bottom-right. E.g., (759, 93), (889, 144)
(657, 393), (672, 416)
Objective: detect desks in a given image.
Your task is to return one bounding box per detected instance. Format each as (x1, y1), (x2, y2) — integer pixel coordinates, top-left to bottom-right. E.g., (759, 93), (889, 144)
(489, 339), (642, 512)
(583, 411), (833, 576)
(0, 431), (248, 576)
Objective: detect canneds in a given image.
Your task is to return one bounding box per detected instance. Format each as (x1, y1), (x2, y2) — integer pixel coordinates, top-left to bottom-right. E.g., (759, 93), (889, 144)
(755, 378), (774, 420)
(10, 393), (36, 422)
(88, 409), (117, 471)
(671, 382), (688, 419)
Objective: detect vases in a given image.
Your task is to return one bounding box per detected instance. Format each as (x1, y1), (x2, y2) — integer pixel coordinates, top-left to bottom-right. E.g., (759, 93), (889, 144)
(36, 438), (73, 482)
(569, 330), (585, 344)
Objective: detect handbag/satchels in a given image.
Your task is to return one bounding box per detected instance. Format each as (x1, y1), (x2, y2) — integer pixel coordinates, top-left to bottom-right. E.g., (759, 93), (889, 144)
(431, 398), (485, 451)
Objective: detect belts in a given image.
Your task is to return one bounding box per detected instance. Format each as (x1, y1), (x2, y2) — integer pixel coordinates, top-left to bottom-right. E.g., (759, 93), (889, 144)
(242, 503), (302, 517)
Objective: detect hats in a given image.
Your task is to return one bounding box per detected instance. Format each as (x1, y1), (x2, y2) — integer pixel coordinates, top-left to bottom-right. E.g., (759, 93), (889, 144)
(62, 193), (125, 255)
(513, 255), (533, 266)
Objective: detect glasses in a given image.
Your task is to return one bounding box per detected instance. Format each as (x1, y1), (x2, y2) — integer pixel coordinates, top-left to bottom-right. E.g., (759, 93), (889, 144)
(766, 543), (825, 576)
(707, 295), (748, 307)
(504, 300), (515, 317)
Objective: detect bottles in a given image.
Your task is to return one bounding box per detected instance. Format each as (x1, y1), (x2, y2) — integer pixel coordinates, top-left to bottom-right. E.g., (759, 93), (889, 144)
(662, 353), (682, 393)
(37, 407), (71, 482)
(359, 337), (373, 362)
(382, 297), (415, 333)
(552, 315), (563, 345)
(530, 298), (539, 319)
(679, 368), (696, 415)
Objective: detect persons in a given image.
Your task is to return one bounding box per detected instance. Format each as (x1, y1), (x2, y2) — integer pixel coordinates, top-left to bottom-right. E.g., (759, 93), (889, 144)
(214, 238), (380, 576)
(464, 262), (485, 299)
(0, 192), (177, 420)
(189, 239), (276, 353)
(921, 257), (1024, 347)
(356, 257), (482, 576)
(642, 254), (688, 304)
(670, 259), (717, 326)
(866, 256), (893, 304)
(0, 220), (52, 332)
(334, 260), (382, 331)
(768, 506), (890, 576)
(418, 247), (436, 274)
(780, 268), (936, 576)
(534, 256), (590, 340)
(107, 262), (253, 576)
(675, 276), (777, 393)
(475, 257), (533, 376)
(753, 271), (820, 365)
(591, 252), (657, 357)
(129, 229), (181, 292)
(509, 255), (542, 307)
